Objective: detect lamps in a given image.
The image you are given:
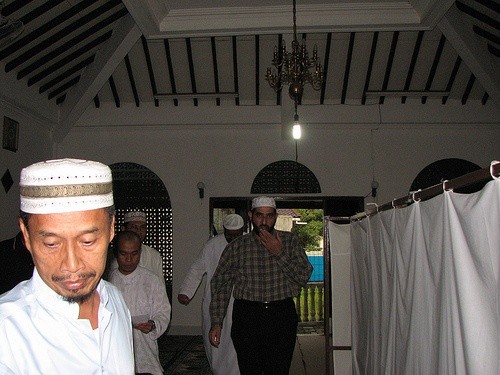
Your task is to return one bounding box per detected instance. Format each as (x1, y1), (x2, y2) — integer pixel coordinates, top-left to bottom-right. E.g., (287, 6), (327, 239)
(153, 92), (239, 98)
(366, 90), (451, 96)
(197, 181), (205, 199)
(370, 181), (379, 197)
(266, 0), (323, 101)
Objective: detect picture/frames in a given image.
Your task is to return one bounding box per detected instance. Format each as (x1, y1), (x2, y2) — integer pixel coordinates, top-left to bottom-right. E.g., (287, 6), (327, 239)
(3, 116), (19, 152)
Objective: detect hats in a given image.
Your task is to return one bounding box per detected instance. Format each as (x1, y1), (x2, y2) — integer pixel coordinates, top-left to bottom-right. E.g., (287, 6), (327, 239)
(19, 158), (113, 214)
(124, 212), (147, 222)
(252, 196), (277, 211)
(223, 214), (244, 230)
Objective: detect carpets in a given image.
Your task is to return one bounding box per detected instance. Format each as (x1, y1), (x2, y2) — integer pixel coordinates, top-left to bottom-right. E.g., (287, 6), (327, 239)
(158, 334), (214, 375)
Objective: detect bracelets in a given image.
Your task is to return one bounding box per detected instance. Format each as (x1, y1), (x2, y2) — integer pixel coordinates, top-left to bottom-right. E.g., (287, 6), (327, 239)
(148, 320), (155, 330)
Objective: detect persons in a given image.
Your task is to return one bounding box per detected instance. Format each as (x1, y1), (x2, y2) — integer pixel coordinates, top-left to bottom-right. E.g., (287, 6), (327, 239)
(108, 211), (162, 277)
(102, 230), (171, 375)
(0, 231), (34, 295)
(0, 158), (135, 375)
(178, 213), (248, 375)
(208, 196), (313, 375)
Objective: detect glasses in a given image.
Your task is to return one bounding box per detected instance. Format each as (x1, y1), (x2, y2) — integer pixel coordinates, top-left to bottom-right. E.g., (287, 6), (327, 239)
(127, 225), (148, 232)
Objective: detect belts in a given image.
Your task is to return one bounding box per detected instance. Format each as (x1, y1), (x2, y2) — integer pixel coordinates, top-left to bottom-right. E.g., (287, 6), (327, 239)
(243, 301), (286, 309)
